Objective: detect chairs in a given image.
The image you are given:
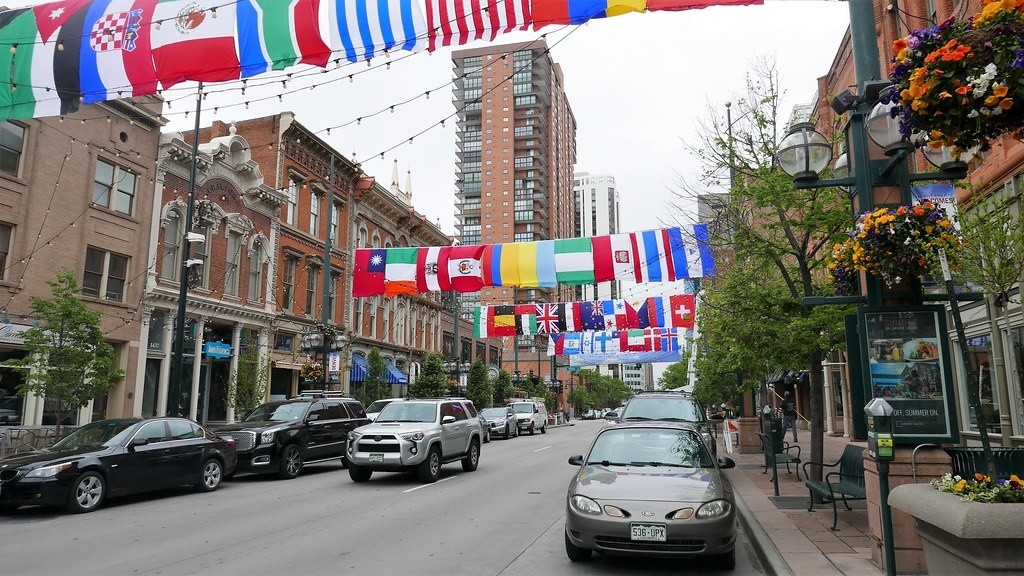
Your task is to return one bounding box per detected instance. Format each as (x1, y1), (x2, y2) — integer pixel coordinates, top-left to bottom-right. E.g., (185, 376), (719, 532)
(147, 426), (162, 442)
(604, 440), (641, 460)
(630, 401), (650, 417)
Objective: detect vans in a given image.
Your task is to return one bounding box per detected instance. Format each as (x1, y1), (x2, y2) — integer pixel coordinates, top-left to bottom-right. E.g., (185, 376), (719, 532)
(506, 400), (548, 436)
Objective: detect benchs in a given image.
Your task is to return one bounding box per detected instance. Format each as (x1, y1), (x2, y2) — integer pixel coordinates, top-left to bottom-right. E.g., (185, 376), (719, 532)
(756, 433), (802, 482)
(803, 444), (867, 531)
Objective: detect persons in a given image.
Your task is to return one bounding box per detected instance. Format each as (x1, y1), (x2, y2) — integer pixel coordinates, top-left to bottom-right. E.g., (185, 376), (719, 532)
(780, 391), (798, 442)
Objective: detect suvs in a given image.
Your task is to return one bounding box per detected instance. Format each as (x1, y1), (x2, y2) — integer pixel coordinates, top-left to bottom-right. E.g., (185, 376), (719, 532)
(345, 396), (483, 484)
(603, 390), (718, 462)
(203, 390), (368, 480)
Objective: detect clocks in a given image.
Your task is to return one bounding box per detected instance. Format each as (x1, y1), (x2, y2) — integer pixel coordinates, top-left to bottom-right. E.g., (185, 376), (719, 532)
(486, 369), (498, 381)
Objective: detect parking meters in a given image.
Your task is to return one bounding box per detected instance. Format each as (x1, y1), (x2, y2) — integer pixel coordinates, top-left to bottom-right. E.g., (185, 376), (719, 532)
(762, 403), (779, 496)
(864, 397), (898, 576)
(712, 404), (718, 439)
(720, 403), (726, 421)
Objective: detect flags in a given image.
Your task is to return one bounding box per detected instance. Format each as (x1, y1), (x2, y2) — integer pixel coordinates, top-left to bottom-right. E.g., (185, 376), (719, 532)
(473, 294), (695, 339)
(352, 223), (716, 297)
(547, 328), (678, 356)
(380, 359), (407, 384)
(0, 0), (764, 121)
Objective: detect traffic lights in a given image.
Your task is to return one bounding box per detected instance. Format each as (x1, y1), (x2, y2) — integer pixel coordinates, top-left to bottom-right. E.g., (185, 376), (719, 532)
(558, 383), (563, 395)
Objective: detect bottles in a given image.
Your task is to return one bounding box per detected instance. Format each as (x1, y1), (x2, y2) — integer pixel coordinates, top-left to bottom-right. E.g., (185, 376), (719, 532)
(870, 339), (903, 361)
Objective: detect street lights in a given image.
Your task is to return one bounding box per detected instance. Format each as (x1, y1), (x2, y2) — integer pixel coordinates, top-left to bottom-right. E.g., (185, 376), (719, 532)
(545, 378), (561, 415)
(777, 96), (981, 309)
(299, 333), (347, 390)
(510, 370), (527, 390)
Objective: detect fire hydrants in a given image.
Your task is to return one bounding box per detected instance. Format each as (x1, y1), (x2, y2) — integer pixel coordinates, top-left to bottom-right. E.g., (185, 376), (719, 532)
(441, 357), (470, 397)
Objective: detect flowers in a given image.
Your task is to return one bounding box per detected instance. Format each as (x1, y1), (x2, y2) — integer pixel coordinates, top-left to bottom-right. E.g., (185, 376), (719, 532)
(930, 472), (1024, 504)
(300, 358), (324, 383)
(879, 0), (1024, 165)
(826, 199), (968, 297)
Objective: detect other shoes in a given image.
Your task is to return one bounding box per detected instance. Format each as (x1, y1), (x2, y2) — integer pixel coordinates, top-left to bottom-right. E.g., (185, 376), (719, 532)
(794, 438), (797, 442)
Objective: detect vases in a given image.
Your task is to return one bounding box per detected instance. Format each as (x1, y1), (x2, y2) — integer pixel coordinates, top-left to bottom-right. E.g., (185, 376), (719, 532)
(887, 483), (1024, 576)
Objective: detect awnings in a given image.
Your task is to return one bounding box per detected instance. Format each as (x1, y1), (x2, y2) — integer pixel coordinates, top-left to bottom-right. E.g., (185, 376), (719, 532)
(350, 353), (369, 381)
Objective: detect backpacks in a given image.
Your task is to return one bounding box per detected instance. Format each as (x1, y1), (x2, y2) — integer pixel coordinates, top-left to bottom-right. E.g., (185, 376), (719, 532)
(786, 398), (795, 412)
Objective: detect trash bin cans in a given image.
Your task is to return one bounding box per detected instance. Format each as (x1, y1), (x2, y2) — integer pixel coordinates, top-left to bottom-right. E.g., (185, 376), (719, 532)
(944, 447), (1024, 481)
(760, 418), (784, 454)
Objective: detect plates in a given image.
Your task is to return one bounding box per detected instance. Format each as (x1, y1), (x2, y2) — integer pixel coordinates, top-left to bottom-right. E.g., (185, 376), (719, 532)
(902, 338), (939, 361)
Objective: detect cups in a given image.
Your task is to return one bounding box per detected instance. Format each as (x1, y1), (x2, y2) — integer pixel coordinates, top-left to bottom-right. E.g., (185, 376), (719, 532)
(885, 355), (892, 361)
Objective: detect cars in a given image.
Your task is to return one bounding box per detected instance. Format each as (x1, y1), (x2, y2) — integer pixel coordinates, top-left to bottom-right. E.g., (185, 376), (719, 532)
(364, 398), (409, 422)
(478, 414), (492, 443)
(478, 407), (519, 440)
(565, 422), (738, 572)
(0, 416), (238, 517)
(581, 406), (626, 420)
